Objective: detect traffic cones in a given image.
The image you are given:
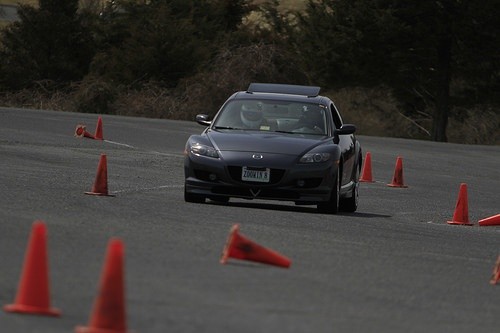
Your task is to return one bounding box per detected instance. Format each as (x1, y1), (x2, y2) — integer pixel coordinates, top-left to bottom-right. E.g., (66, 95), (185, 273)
(478, 214), (500, 226)
(445, 183), (476, 226)
(221, 225), (292, 268)
(386, 156), (409, 188)
(92, 117), (105, 141)
(74, 126), (96, 139)
(359, 149), (377, 182)
(72, 237), (135, 333)
(82, 154), (117, 198)
(4, 217), (64, 319)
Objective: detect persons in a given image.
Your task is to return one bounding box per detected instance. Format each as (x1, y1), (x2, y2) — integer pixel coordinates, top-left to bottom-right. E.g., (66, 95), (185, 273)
(230, 101), (268, 129)
(291, 105), (325, 134)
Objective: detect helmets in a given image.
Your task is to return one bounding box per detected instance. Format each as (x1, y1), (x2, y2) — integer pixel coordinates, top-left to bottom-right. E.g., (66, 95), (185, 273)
(241, 102), (262, 127)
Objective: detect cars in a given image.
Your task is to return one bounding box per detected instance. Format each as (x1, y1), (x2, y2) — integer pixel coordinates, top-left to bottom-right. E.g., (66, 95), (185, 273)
(182, 82), (366, 211)
